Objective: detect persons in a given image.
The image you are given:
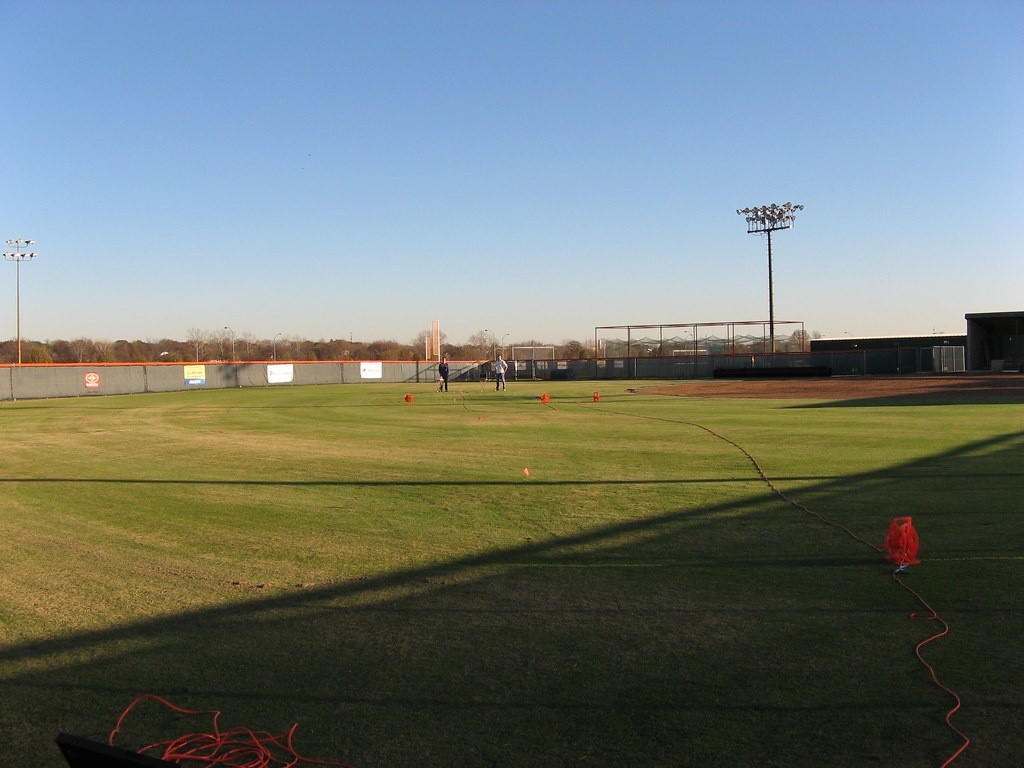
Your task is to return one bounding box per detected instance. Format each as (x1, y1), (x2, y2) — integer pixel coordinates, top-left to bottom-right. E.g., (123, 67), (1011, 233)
(438, 357), (449, 392)
(494, 354), (508, 391)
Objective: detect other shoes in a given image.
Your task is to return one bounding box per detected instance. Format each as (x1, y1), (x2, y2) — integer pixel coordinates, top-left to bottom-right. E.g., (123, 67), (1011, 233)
(503, 389), (506, 391)
(445, 389), (448, 392)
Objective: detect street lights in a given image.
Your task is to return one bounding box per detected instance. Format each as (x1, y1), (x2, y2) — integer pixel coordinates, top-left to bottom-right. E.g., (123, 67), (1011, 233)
(225, 327), (235, 360)
(485, 329), (509, 360)
(3, 238), (37, 366)
(736, 200), (803, 355)
(273, 333), (281, 361)
